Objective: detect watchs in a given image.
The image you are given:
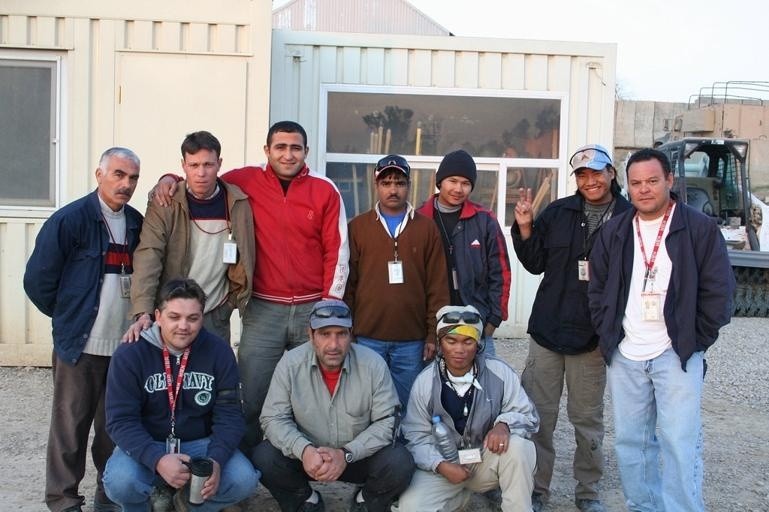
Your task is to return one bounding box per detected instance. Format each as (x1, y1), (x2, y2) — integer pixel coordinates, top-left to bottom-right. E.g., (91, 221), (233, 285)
(341, 447), (354, 465)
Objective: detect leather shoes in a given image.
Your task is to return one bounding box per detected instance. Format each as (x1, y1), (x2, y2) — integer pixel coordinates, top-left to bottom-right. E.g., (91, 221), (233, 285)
(576, 499), (606, 512)
(297, 490), (326, 511)
(351, 488), (367, 512)
(532, 500), (543, 512)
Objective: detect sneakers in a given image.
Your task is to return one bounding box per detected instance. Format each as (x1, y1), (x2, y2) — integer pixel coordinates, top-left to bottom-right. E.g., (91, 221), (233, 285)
(173, 489), (189, 512)
(152, 487), (174, 512)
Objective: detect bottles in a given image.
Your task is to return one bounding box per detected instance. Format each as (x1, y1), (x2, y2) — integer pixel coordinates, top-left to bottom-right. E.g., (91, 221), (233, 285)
(432, 415), (461, 464)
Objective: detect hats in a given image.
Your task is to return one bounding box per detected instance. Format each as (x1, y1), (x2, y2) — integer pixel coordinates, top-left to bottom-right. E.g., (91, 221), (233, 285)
(436, 150), (478, 193)
(435, 304), (485, 336)
(374, 155), (411, 179)
(570, 145), (614, 176)
(309, 300), (354, 329)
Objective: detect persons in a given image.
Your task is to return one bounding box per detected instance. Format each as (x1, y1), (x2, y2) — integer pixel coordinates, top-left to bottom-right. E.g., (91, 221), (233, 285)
(23, 147), (144, 511)
(414, 150), (511, 357)
(121, 130), (255, 347)
(510, 145), (632, 512)
(586, 147), (735, 511)
(347, 154), (451, 417)
(103, 279), (261, 512)
(250, 301), (415, 511)
(148, 122), (351, 448)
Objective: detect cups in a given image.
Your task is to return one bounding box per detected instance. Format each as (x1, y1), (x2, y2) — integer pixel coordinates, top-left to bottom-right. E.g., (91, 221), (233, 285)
(182, 456), (213, 507)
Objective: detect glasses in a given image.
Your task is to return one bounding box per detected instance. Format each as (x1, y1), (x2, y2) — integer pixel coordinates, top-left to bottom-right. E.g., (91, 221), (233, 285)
(436, 311), (485, 329)
(377, 159), (410, 173)
(309, 306), (353, 327)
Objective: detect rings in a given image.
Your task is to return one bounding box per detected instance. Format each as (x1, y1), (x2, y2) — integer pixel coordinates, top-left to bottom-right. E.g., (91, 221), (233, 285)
(499, 443), (504, 447)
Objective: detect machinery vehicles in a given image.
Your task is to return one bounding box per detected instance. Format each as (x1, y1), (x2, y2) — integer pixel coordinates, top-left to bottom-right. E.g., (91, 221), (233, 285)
(653, 133), (769, 318)
